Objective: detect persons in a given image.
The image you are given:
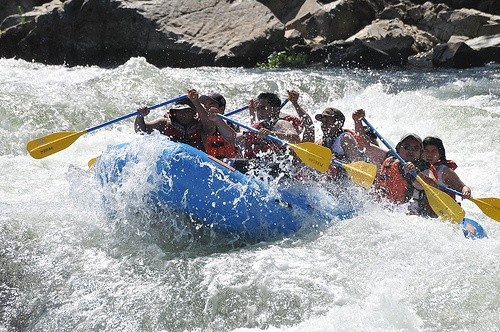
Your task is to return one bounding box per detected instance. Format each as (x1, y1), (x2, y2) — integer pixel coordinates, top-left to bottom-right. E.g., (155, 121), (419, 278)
(133, 89), (470, 216)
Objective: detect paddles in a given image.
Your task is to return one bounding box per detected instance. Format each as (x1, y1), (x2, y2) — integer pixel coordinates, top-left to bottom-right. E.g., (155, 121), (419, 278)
(280, 94), (296, 108)
(359, 114), (465, 225)
(329, 152), (378, 190)
(433, 179), (500, 222)
(212, 110), (332, 172)
(27, 92), (197, 159)
(222, 103), (251, 119)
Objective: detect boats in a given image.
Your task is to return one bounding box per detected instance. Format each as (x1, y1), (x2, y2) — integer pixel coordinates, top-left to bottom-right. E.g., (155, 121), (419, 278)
(96, 132), (358, 242)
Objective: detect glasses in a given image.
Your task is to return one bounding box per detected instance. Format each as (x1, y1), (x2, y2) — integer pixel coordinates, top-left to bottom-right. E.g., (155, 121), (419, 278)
(400, 145), (421, 151)
(255, 106), (272, 111)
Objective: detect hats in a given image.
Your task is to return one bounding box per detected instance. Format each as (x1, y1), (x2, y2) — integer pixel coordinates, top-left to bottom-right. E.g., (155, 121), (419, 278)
(197, 93), (226, 109)
(363, 126), (379, 145)
(315, 107), (345, 127)
(169, 98), (196, 116)
(395, 132), (423, 150)
